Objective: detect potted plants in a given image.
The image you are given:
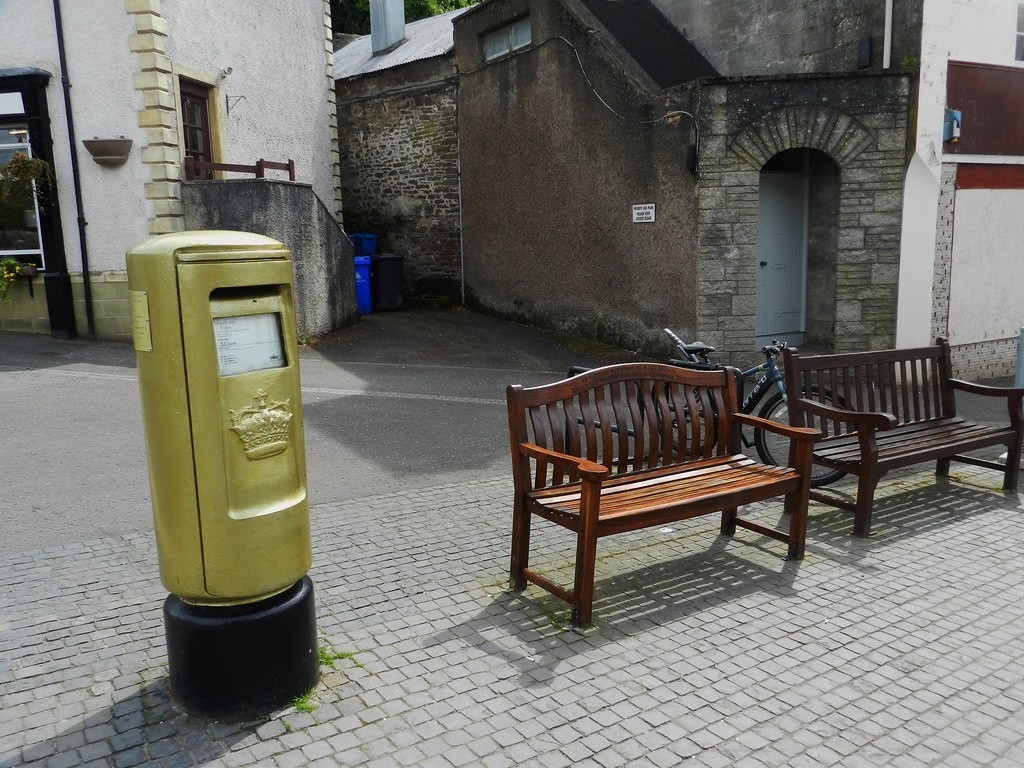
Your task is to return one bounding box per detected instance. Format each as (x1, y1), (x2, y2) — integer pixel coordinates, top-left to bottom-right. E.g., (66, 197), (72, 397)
(0, 151), (57, 215)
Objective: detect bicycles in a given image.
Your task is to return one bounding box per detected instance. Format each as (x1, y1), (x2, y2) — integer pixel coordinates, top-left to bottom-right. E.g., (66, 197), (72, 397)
(650, 337), (860, 489)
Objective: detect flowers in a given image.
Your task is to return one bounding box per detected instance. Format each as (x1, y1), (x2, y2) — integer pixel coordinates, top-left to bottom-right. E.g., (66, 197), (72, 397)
(0, 256), (22, 304)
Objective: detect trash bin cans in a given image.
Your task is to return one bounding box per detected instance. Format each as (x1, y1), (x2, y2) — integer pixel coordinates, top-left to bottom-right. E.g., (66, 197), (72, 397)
(126, 229), (320, 714)
(371, 252), (407, 311)
(351, 232), (377, 314)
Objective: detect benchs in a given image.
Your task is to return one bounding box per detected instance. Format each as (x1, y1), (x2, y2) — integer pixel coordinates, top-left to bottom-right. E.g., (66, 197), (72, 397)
(782, 336), (1024, 539)
(506, 362), (826, 628)
